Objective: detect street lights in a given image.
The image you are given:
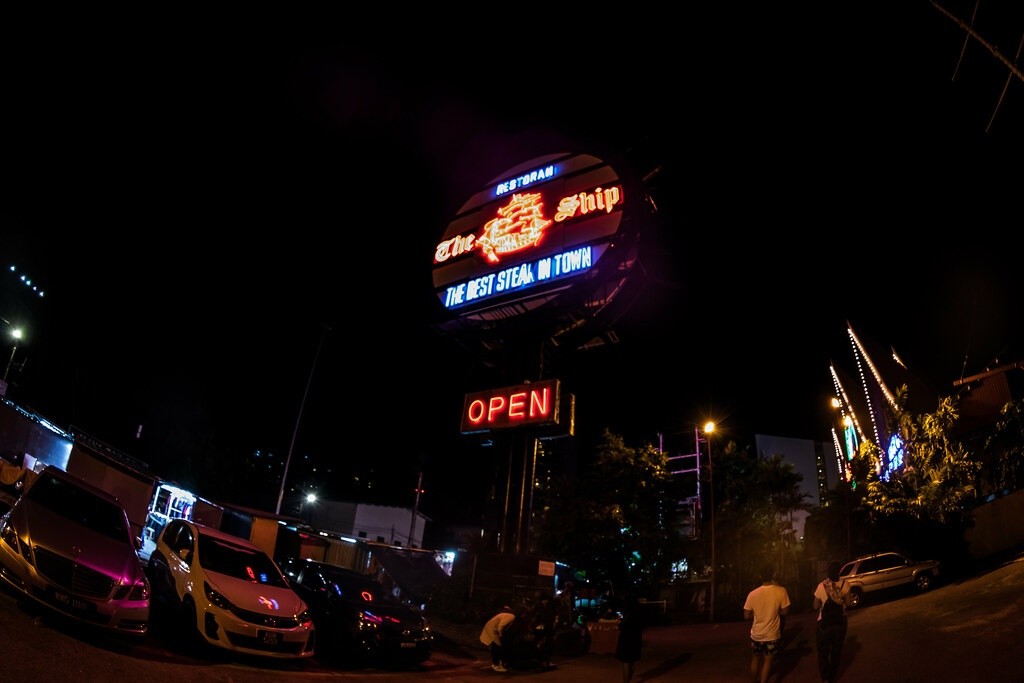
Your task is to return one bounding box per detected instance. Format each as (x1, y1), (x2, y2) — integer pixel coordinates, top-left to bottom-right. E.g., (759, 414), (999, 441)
(702, 419), (722, 624)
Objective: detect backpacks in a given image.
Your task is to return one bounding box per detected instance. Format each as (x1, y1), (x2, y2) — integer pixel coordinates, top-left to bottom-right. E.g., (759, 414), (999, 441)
(821, 579), (845, 624)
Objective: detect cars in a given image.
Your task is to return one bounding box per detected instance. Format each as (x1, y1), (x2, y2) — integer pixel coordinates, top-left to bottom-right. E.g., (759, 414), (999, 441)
(0, 461), (152, 636)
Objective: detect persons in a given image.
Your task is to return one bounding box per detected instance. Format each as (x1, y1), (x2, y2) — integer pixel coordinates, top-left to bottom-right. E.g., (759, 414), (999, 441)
(480, 612), (524, 674)
(742, 565), (791, 683)
(813, 560), (853, 683)
(613, 590), (643, 683)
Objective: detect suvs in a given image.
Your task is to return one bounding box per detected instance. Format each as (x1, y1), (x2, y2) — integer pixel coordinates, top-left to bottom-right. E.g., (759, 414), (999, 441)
(837, 551), (941, 607)
(283, 558), (440, 672)
(145, 516), (320, 661)
